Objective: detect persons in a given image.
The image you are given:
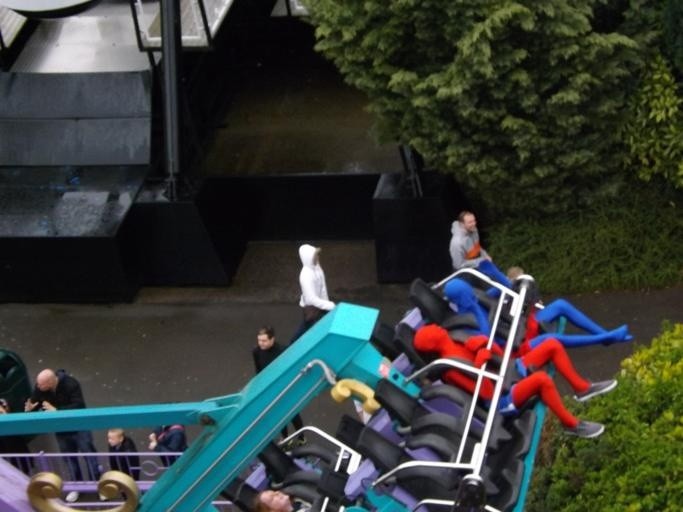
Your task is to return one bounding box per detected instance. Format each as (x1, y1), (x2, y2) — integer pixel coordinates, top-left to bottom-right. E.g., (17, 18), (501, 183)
(443, 277), (635, 357)
(24, 367), (100, 482)
(413, 323), (619, 440)
(252, 325), (306, 452)
(448, 210), (492, 269)
(298, 244), (336, 325)
(0, 398), (34, 476)
(254, 489), (313, 512)
(147, 424), (188, 467)
(106, 428), (140, 501)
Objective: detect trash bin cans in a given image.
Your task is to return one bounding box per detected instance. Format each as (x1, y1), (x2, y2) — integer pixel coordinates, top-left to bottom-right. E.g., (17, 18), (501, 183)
(0, 349), (38, 446)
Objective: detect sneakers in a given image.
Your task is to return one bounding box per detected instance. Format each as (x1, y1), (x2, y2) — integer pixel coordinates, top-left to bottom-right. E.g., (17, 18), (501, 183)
(561, 421), (603, 440)
(574, 378), (617, 402)
